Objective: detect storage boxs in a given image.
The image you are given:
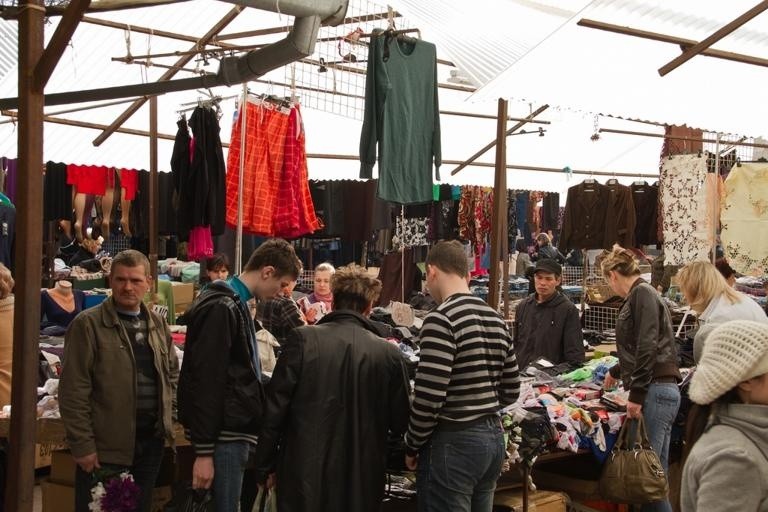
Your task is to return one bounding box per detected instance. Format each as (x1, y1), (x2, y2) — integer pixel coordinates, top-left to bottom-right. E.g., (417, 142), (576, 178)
(164, 279), (194, 314)
(39, 450), (94, 512)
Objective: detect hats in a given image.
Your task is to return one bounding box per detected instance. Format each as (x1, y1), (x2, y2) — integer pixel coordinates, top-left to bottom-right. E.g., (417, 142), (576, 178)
(688, 318), (767, 408)
(532, 257), (563, 277)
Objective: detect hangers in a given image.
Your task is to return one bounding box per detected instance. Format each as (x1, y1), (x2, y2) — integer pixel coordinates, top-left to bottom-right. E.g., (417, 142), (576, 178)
(581, 170), (647, 195)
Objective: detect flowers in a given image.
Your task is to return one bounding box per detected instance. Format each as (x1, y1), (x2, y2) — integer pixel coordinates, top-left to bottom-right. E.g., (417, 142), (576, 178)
(87, 467), (145, 512)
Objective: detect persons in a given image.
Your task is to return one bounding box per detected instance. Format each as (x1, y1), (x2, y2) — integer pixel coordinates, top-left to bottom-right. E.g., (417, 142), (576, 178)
(677, 254), (768, 512)
(512, 257), (585, 375)
(600, 245), (682, 512)
(53, 167), (137, 241)
(178, 239), (335, 511)
(0, 263), (19, 415)
(39, 279), (85, 330)
(532, 234), (566, 266)
(403, 242), (520, 512)
(57, 249), (179, 511)
(257, 262), (409, 511)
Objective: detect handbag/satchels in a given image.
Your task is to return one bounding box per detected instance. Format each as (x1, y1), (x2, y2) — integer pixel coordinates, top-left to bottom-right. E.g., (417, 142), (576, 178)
(598, 410), (670, 505)
